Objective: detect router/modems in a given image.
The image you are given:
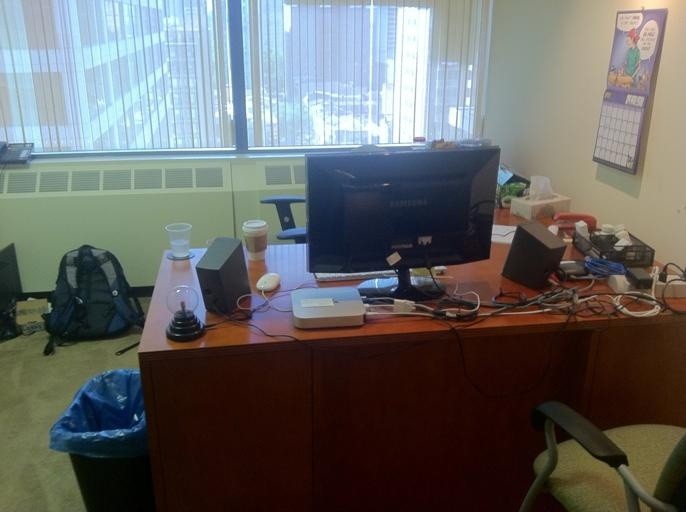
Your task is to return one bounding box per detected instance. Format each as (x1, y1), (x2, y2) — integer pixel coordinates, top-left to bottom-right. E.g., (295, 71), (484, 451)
(290, 286), (366, 329)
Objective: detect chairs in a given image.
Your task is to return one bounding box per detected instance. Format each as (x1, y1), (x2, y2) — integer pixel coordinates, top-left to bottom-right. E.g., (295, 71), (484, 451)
(518, 399), (686, 511)
(259, 195), (307, 243)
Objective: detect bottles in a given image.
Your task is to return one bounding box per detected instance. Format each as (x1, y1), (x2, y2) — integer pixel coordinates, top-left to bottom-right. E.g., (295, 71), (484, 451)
(614, 224), (633, 245)
(600, 224), (614, 237)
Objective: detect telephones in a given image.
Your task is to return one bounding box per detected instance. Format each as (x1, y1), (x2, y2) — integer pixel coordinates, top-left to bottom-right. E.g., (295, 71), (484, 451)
(1, 142), (34, 165)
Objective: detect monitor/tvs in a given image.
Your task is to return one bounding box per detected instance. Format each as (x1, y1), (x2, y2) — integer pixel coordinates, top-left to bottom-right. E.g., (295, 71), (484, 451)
(304, 144), (501, 302)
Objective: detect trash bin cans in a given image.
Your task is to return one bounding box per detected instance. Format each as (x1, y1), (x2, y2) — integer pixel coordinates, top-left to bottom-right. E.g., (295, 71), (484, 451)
(49, 368), (155, 512)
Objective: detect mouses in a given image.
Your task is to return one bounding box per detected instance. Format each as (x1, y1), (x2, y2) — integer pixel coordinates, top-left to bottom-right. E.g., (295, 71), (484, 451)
(257, 273), (280, 291)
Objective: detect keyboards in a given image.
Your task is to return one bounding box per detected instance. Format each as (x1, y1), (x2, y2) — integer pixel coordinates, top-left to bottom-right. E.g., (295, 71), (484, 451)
(313, 269), (397, 282)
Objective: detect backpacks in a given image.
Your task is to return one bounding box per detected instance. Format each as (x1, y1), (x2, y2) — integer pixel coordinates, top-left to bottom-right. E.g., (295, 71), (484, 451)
(42, 245), (145, 355)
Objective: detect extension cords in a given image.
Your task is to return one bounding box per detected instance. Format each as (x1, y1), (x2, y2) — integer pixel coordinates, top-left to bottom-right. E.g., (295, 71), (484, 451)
(609, 273), (686, 299)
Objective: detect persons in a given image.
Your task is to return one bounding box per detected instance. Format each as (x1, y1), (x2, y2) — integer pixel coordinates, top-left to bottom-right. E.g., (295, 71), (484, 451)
(619, 28), (641, 79)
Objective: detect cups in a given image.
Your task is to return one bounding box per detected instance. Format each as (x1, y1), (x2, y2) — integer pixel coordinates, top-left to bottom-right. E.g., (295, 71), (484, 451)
(166, 223), (192, 257)
(243, 221), (268, 261)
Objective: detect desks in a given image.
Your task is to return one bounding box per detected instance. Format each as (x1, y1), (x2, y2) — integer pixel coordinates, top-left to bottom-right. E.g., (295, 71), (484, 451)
(137, 210), (685, 511)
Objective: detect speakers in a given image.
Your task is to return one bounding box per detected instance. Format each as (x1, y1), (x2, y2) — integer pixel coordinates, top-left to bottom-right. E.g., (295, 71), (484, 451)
(501, 221), (567, 290)
(195, 238), (251, 313)
